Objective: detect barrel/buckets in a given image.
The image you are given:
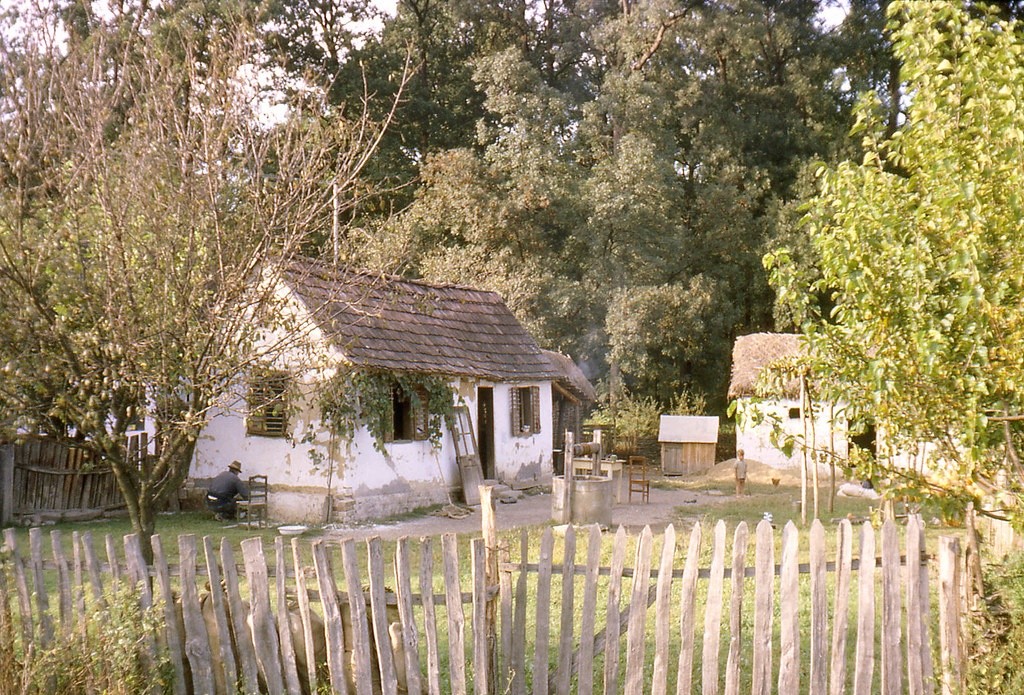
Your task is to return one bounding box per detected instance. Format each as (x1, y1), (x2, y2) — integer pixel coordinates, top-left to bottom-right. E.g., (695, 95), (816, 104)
(793, 501), (802, 513)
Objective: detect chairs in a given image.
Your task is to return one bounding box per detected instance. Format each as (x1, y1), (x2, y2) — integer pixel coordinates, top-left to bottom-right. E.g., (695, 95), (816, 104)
(236, 474), (268, 531)
(629, 456), (650, 504)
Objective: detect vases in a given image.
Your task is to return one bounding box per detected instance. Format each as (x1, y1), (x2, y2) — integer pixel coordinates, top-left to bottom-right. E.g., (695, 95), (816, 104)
(521, 425), (529, 431)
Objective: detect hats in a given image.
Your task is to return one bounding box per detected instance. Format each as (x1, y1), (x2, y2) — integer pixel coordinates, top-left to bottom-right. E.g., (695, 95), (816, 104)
(228, 460), (242, 473)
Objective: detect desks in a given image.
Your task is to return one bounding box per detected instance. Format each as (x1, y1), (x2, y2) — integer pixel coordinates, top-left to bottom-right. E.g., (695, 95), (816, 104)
(573, 458), (626, 503)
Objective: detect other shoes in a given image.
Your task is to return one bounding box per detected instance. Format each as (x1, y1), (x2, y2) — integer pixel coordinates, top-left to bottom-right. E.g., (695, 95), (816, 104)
(222, 519), (236, 524)
(217, 515), (224, 521)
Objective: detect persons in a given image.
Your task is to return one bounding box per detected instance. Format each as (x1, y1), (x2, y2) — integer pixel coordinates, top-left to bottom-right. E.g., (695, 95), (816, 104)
(734, 449), (747, 499)
(207, 460), (248, 520)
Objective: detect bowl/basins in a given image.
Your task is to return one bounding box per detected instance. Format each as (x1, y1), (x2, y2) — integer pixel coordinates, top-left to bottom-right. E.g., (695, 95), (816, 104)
(277, 526), (307, 534)
